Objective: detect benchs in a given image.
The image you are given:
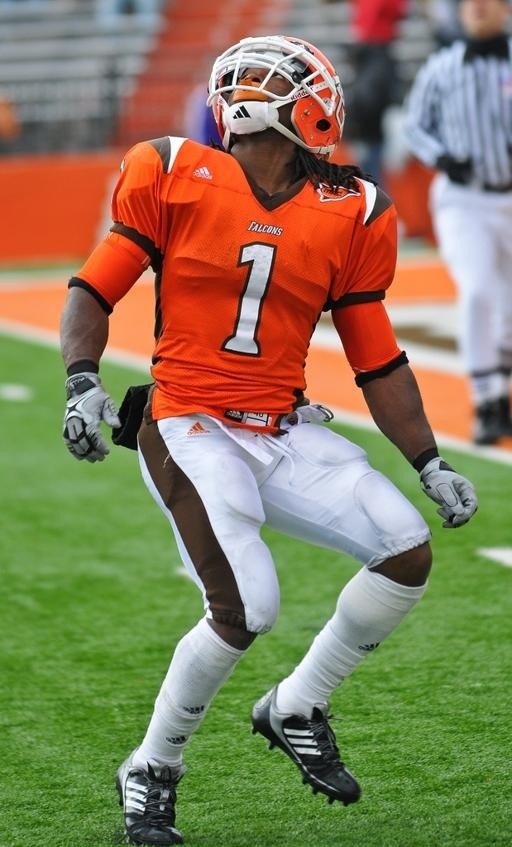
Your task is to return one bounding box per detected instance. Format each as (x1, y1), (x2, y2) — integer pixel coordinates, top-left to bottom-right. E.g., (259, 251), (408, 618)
(268, 1), (465, 120)
(0, 3), (171, 132)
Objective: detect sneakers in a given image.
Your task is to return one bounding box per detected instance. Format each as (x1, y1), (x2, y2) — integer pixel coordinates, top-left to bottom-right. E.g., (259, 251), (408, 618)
(472, 400), (499, 444)
(496, 397), (511, 436)
(114, 747), (187, 845)
(250, 684), (362, 808)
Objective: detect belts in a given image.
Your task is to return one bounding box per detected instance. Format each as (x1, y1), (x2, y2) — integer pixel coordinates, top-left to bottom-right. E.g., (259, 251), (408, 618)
(480, 181), (512, 192)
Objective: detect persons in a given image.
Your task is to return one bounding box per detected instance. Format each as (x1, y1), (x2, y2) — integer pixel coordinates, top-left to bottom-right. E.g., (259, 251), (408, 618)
(333, 0), (417, 44)
(59, 33), (478, 845)
(396, 0), (510, 447)
(338, 40), (404, 240)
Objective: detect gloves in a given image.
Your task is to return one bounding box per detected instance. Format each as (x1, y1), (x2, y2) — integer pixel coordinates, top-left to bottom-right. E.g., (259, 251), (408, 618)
(433, 154), (474, 187)
(59, 371), (123, 465)
(420, 456), (478, 529)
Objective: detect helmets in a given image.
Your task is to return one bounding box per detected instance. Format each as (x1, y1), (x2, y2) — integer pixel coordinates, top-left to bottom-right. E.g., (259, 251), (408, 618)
(208, 32), (347, 157)
